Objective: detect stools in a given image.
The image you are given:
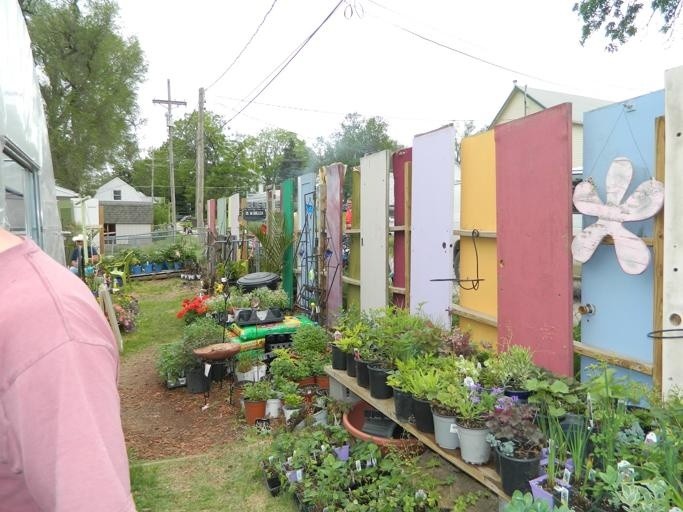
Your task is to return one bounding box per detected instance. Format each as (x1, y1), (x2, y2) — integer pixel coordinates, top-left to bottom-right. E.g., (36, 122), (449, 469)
(200, 356), (234, 404)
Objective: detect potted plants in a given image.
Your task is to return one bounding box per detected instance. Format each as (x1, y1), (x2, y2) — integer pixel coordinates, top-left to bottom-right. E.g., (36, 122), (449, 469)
(215, 259), (246, 288)
(208, 318), (227, 392)
(163, 326), (209, 393)
(116, 244), (196, 286)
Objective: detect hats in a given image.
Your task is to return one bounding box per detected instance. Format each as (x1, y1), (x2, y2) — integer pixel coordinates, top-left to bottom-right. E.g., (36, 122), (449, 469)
(72, 234), (89, 242)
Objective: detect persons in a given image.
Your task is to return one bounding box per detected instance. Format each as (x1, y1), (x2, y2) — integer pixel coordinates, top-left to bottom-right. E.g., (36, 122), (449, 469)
(0, 224), (136, 512)
(68, 234), (100, 275)
